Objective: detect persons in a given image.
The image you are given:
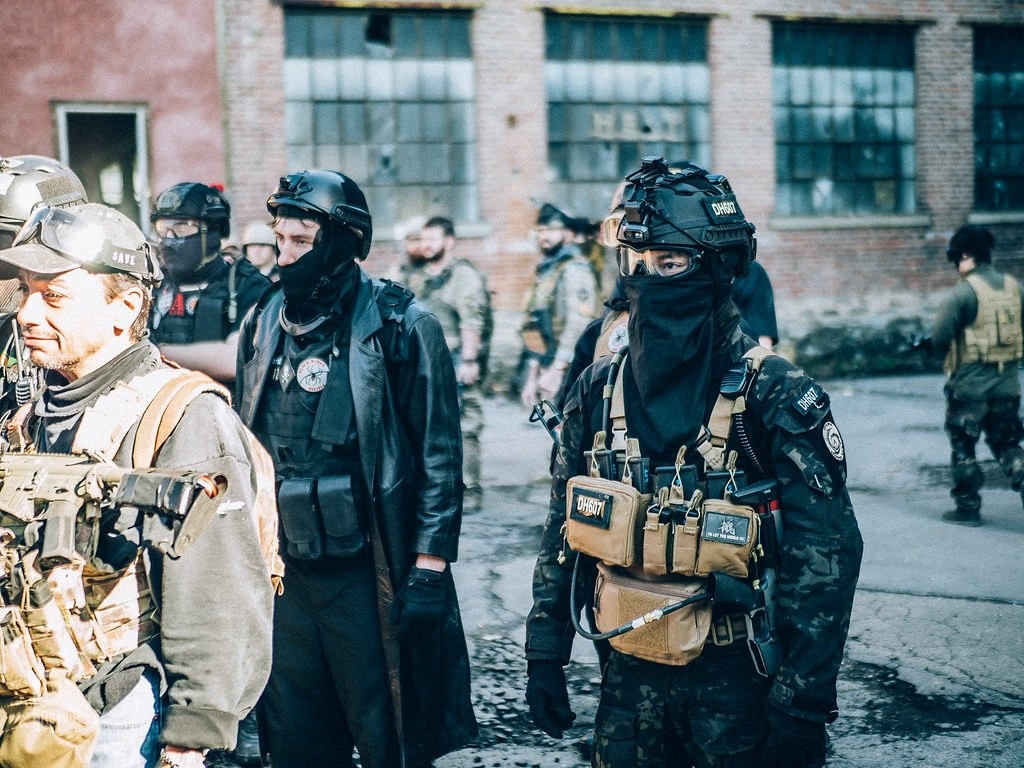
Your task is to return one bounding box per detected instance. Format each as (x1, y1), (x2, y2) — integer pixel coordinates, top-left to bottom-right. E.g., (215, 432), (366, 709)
(0, 154), (88, 398)
(526, 155), (864, 768)
(0, 204), (278, 768)
(236, 168), (480, 768)
(925, 222), (1024, 526)
(149, 162), (781, 515)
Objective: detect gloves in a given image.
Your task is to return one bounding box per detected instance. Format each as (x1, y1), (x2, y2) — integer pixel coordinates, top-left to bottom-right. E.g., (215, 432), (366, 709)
(751, 705), (826, 768)
(388, 566), (447, 644)
(525, 660), (576, 739)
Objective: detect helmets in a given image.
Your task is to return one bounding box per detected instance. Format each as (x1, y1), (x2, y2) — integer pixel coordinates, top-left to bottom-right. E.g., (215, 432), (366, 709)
(946, 226), (993, 259)
(149, 182), (231, 238)
(266, 170), (372, 261)
(618, 156), (757, 277)
(241, 222), (278, 255)
(610, 166), (685, 218)
(0, 155), (89, 238)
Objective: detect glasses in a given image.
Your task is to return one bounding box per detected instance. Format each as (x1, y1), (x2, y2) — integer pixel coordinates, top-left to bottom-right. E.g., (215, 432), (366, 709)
(600, 217), (629, 247)
(156, 221), (201, 240)
(11, 205), (149, 273)
(619, 245), (702, 279)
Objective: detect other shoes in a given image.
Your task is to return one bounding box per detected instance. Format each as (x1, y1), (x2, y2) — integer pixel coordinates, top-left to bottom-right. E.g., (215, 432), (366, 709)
(234, 709), (263, 766)
(462, 488), (482, 514)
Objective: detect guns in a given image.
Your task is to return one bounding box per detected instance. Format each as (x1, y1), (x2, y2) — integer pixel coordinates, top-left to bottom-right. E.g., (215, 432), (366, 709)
(705, 479), (786, 679)
(1, 452), (232, 572)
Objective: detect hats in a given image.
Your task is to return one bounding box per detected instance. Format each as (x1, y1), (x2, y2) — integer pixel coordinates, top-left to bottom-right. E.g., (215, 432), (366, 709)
(0, 202), (164, 288)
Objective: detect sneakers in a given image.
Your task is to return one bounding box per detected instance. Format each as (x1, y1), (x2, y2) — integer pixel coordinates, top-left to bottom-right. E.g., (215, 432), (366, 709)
(941, 509), (981, 527)
